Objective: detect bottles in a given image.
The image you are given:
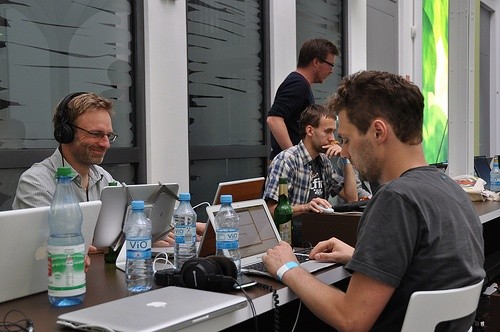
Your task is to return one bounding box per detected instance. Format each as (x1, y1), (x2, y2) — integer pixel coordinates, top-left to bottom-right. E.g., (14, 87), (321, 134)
(172, 192), (198, 269)
(124, 199), (154, 293)
(274, 178), (294, 251)
(104, 181), (122, 262)
(214, 194), (241, 275)
(489, 156), (500, 193)
(48, 167), (87, 307)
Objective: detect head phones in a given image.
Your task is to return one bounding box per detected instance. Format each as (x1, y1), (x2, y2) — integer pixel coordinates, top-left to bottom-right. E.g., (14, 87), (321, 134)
(54, 92), (85, 144)
(153, 255), (237, 292)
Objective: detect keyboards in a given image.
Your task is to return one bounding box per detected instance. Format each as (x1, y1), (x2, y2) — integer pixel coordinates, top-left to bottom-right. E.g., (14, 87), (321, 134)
(332, 199), (367, 212)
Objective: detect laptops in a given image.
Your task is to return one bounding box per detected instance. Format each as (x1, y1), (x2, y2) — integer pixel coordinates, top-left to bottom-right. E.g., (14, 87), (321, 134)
(205, 198), (337, 280)
(56, 282), (249, 332)
(152, 177), (264, 258)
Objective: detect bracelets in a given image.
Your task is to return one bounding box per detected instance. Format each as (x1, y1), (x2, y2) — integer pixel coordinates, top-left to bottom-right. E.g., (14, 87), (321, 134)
(276, 261), (299, 281)
(341, 159), (350, 164)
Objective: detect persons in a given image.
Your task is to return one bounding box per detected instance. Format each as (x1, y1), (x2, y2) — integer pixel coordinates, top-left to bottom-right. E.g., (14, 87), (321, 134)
(266, 38), (339, 164)
(263, 103), (359, 218)
(336, 128), (373, 205)
(12, 93), (206, 247)
(261, 70), (486, 332)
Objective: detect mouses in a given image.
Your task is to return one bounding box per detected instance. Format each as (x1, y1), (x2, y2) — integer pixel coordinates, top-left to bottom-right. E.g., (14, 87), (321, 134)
(313, 203), (334, 213)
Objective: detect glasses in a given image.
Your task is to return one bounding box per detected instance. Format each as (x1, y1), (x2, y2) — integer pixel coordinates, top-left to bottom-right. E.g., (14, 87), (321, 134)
(322, 59), (335, 68)
(67, 123), (119, 142)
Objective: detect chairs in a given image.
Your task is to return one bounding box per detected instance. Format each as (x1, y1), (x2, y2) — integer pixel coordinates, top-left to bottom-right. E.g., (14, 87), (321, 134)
(398, 280), (486, 332)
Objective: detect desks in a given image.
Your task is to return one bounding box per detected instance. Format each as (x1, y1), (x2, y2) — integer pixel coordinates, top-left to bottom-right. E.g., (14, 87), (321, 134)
(0, 194), (500, 332)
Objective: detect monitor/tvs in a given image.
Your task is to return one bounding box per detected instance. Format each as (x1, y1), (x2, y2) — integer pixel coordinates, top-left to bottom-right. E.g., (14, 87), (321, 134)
(473, 158), (492, 190)
(0, 200), (102, 302)
(94, 183), (179, 262)
